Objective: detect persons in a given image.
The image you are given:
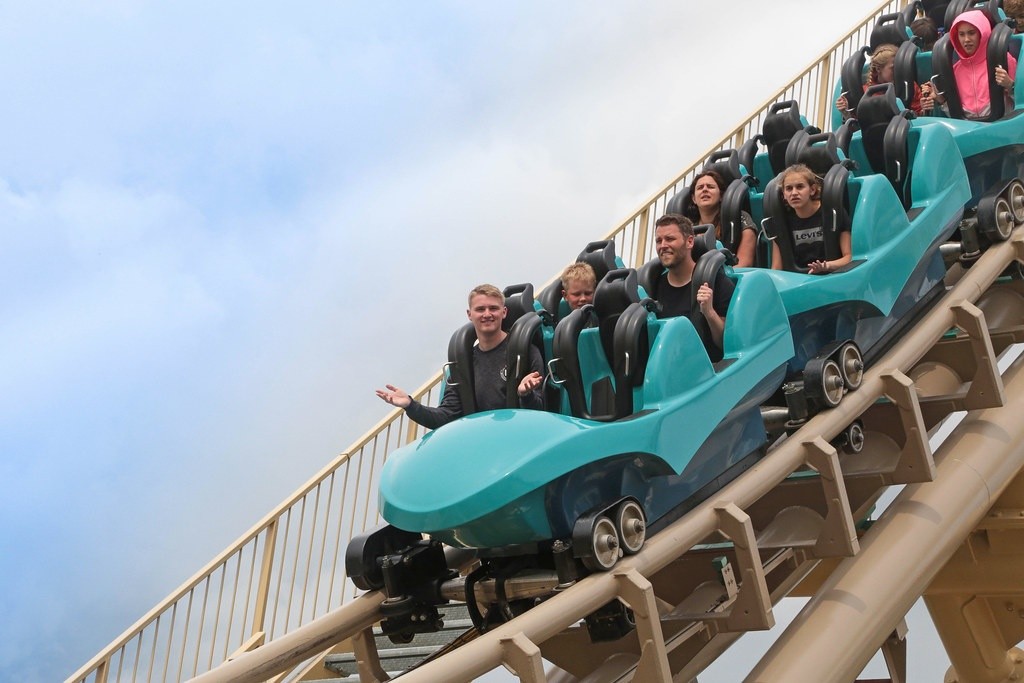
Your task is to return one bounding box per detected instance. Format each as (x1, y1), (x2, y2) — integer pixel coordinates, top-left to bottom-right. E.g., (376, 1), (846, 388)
(1001, 0), (1024, 34)
(644, 213), (736, 363)
(921, 9), (1022, 120)
(770, 164), (852, 275)
(910, 18), (943, 51)
(685, 171), (759, 268)
(835, 43), (937, 126)
(373, 284), (548, 431)
(553, 261), (601, 328)
